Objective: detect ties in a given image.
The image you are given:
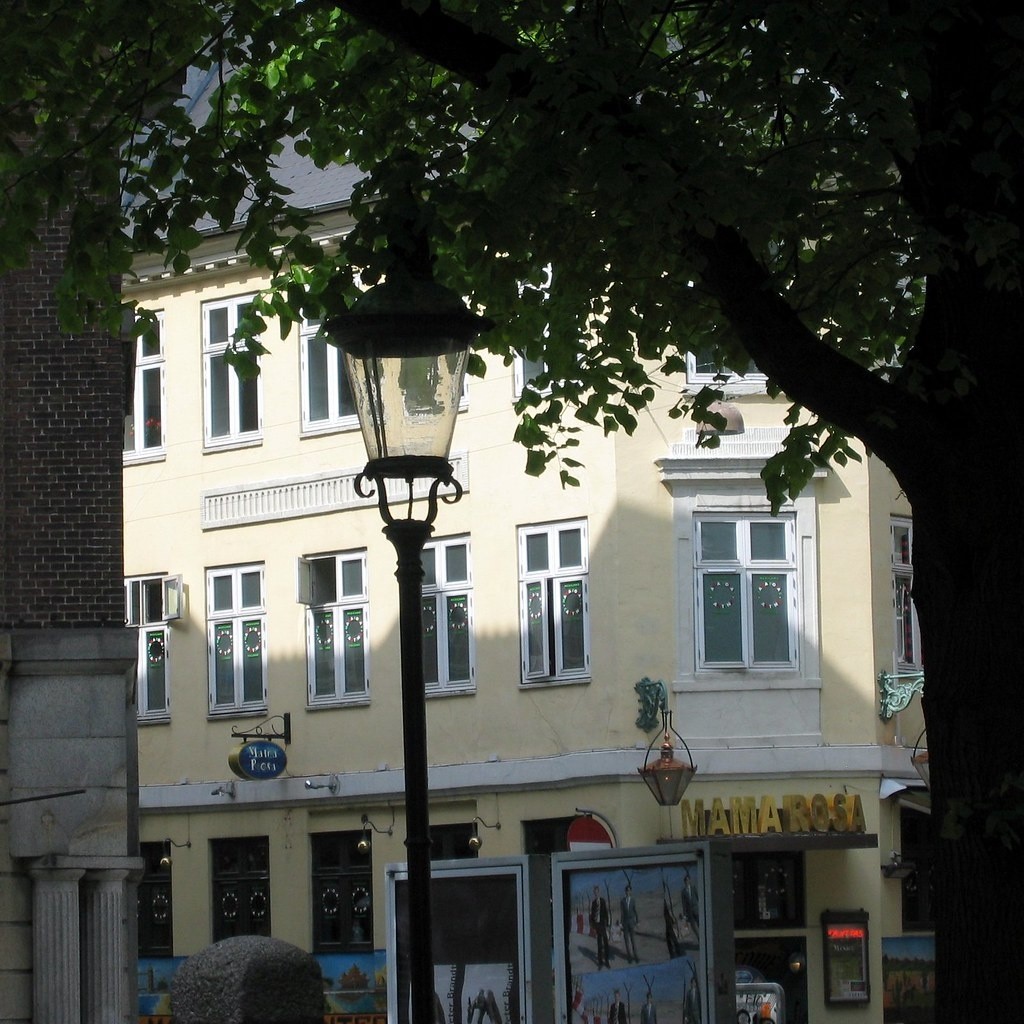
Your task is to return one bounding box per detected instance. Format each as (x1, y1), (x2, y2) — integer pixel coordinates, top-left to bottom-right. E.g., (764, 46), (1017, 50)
(614, 1007), (617, 1024)
(626, 896), (629, 907)
(692, 990), (694, 1004)
(688, 887), (689, 897)
(647, 1005), (650, 1016)
(595, 900), (600, 921)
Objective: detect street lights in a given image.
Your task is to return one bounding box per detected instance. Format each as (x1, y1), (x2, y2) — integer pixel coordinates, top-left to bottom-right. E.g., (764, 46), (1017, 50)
(320, 212), (489, 1024)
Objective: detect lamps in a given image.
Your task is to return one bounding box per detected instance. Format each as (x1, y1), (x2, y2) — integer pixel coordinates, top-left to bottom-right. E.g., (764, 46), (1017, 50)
(357, 821), (393, 853)
(877, 671), (931, 792)
(304, 773), (341, 796)
(468, 816), (500, 850)
(159, 838), (192, 867)
(212, 780), (236, 798)
(633, 676), (698, 806)
(881, 852), (914, 879)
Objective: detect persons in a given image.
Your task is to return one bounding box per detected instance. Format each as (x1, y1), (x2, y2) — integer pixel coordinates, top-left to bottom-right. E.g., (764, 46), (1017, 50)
(592, 876), (701, 969)
(609, 977), (701, 1024)
